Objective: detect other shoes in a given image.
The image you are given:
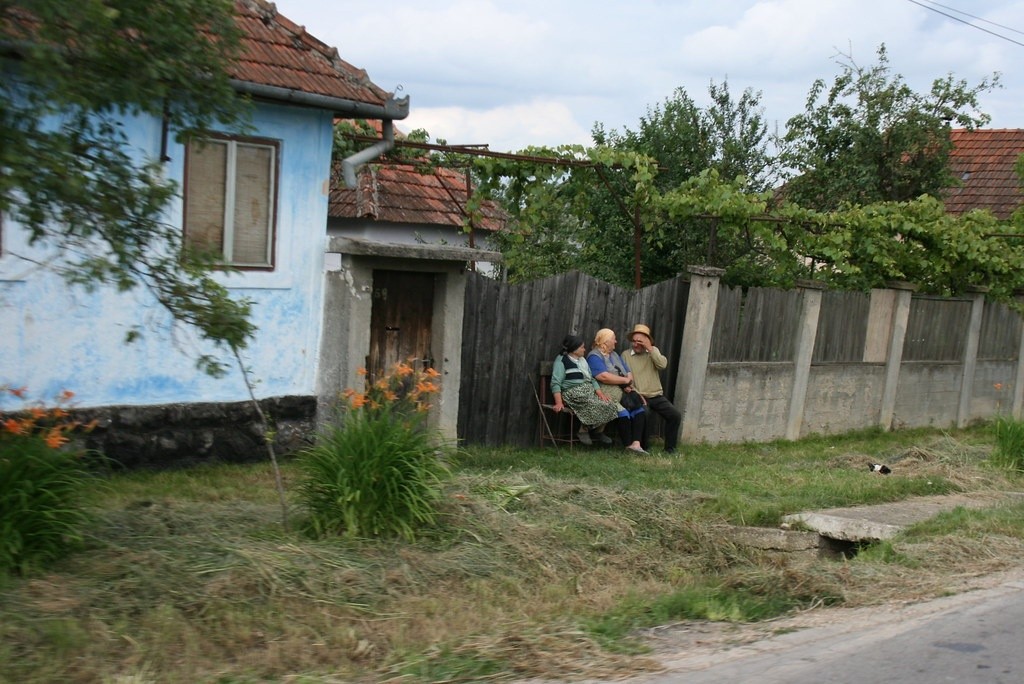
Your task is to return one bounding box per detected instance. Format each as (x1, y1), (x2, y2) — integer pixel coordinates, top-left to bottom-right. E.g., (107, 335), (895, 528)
(625, 446), (649, 456)
(593, 432), (612, 444)
(576, 432), (592, 445)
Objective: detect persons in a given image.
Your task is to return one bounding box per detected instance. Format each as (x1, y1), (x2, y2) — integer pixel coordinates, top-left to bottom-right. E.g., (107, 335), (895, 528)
(622, 323), (682, 458)
(552, 338), (618, 446)
(587, 328), (653, 458)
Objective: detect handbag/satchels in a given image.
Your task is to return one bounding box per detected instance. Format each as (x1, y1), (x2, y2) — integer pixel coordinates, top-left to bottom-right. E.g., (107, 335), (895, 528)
(620, 390), (644, 412)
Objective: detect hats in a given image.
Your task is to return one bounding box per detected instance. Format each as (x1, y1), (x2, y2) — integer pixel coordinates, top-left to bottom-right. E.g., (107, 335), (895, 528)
(627, 324), (654, 345)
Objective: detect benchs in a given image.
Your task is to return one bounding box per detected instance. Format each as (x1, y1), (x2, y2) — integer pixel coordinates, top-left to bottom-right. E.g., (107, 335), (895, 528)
(539, 360), (662, 454)
